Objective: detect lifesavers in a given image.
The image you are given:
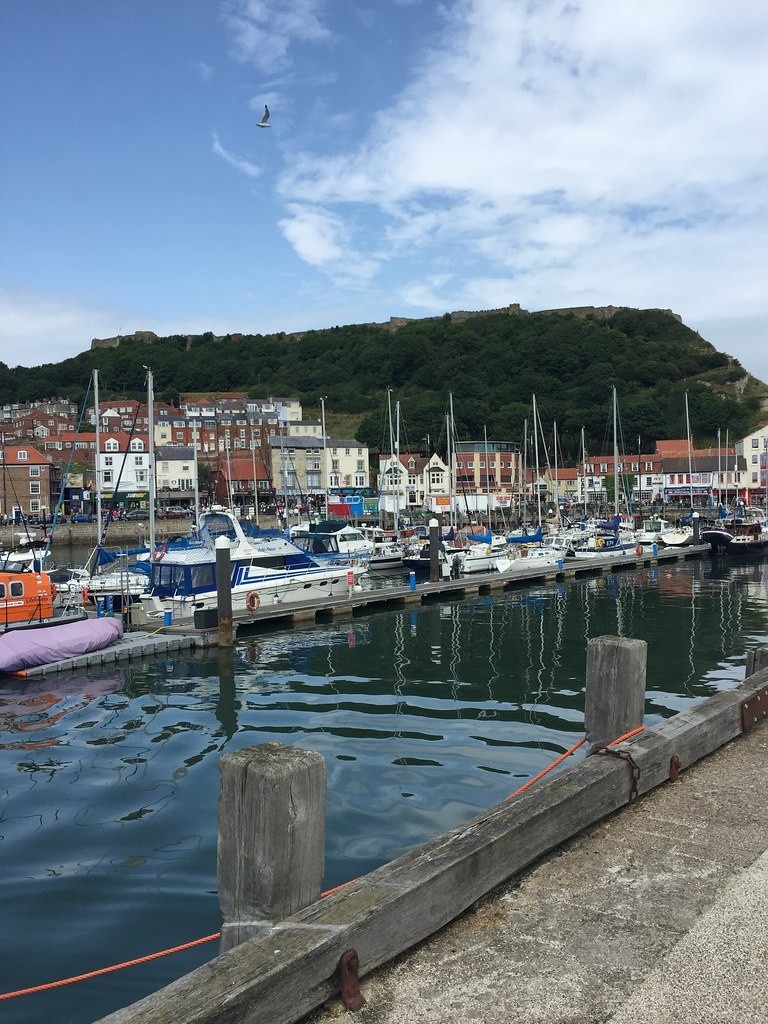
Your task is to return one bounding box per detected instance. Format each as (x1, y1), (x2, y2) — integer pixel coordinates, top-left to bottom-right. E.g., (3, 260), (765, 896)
(152, 545), (167, 562)
(51, 585), (57, 602)
(245, 591), (260, 612)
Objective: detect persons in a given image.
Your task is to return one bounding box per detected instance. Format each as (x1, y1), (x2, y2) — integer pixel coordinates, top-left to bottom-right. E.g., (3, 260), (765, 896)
(452, 555), (461, 579)
(57, 509), (61, 524)
(0, 512), (27, 526)
(113, 508), (124, 521)
(236, 493), (323, 514)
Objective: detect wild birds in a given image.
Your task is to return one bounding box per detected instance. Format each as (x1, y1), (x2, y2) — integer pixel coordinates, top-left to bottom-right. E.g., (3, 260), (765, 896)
(255, 104), (272, 128)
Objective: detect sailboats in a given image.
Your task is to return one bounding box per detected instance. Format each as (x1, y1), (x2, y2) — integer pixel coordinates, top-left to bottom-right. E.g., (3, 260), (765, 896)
(0, 362), (768, 646)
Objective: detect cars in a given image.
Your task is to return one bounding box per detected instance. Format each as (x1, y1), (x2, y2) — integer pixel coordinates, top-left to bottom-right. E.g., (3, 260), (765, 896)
(126, 509), (150, 520)
(71, 513), (100, 523)
(158, 510), (184, 520)
(166, 506), (194, 517)
(28, 513), (67, 525)
